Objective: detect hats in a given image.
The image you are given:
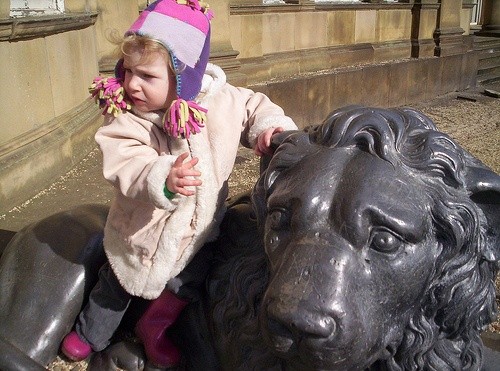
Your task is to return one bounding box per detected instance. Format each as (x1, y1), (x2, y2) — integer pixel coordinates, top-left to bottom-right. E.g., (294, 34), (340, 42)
(90, 0), (213, 138)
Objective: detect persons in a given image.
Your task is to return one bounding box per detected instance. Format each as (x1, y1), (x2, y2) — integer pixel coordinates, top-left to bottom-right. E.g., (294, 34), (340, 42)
(60, 0), (299, 369)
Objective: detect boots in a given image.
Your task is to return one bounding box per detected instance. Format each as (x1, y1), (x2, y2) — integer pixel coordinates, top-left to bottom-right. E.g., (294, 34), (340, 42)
(61, 331), (88, 362)
(132, 288), (189, 365)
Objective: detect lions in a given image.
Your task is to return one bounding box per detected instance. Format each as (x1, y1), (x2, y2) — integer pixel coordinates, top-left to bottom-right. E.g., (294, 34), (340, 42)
(0, 103), (500, 371)
